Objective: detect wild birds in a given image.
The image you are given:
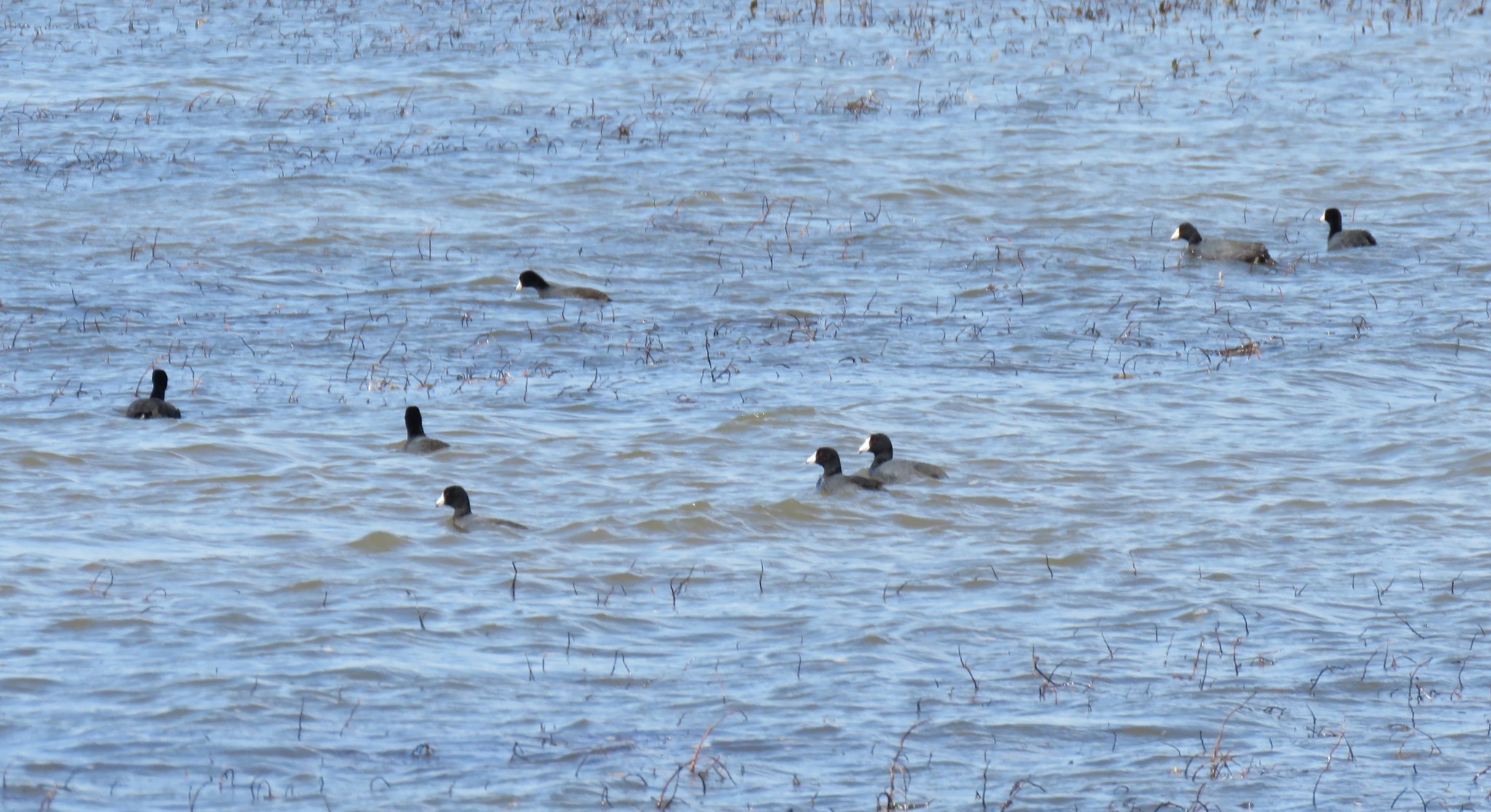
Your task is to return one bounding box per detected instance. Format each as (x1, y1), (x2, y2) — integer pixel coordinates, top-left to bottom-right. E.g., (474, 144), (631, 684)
(400, 405), (452, 455)
(1319, 207), (1378, 252)
(516, 270), (612, 303)
(433, 484), (529, 539)
(125, 368), (181, 419)
(857, 433), (949, 486)
(801, 446), (887, 495)
(1169, 220), (1276, 266)
